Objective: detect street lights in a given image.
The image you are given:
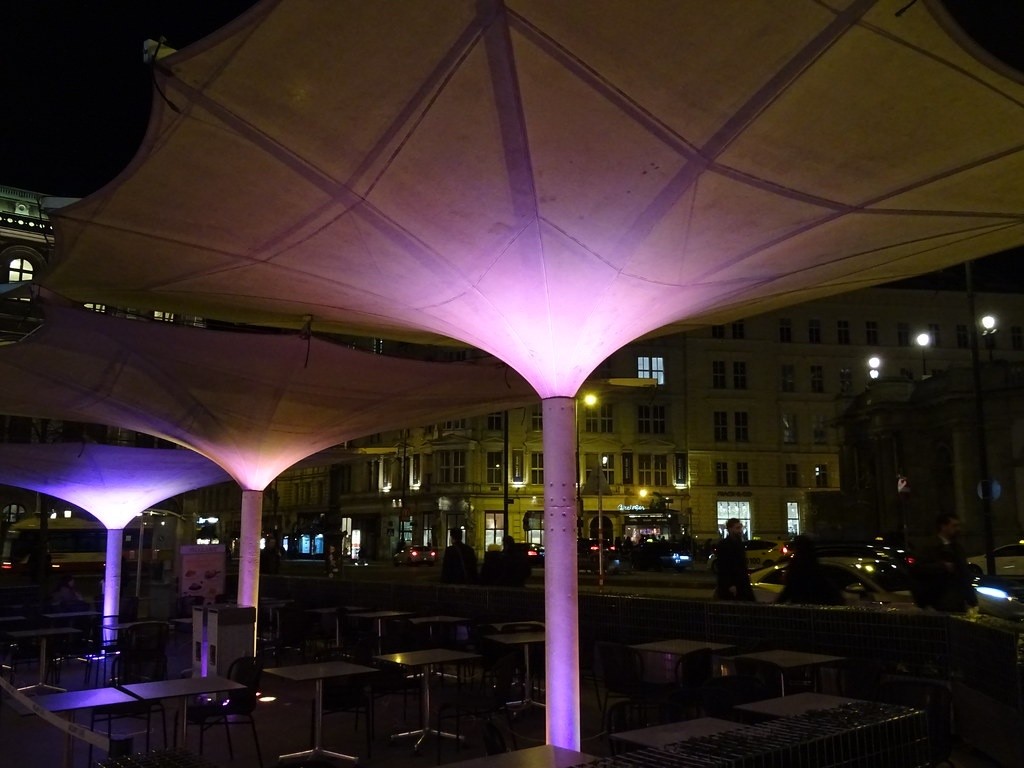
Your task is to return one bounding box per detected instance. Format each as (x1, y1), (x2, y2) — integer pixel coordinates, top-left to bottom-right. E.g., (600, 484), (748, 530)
(574, 391), (598, 537)
(914, 313), (999, 582)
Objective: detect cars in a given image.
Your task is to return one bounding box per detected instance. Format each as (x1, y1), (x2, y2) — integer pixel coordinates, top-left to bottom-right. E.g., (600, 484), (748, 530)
(749, 562), (892, 609)
(523, 542), (542, 563)
(393, 544), (436, 567)
(577, 538), (616, 559)
(707, 540), (795, 574)
(966, 540), (1024, 582)
(814, 543), (919, 590)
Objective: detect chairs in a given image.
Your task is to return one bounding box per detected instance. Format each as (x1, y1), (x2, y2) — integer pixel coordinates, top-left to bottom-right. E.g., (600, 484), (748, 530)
(0, 601), (848, 768)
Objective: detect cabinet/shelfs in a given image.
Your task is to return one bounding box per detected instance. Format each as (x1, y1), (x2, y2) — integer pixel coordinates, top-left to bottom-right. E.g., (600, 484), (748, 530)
(192, 606), (257, 679)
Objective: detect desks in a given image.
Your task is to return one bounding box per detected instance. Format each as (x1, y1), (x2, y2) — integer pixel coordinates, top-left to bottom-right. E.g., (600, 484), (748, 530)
(628, 640), (735, 713)
(304, 607), (367, 658)
(0, 616), (27, 622)
(120, 677), (248, 748)
(394, 616), (471, 679)
(6, 627), (83, 693)
(611, 716), (750, 748)
(346, 610), (414, 655)
(436, 744), (602, 768)
(99, 620), (171, 681)
(722, 649), (845, 697)
(41, 611), (103, 618)
(174, 618), (193, 623)
(262, 661), (380, 765)
(485, 632), (545, 714)
(5, 686), (138, 768)
(734, 693), (866, 717)
(260, 596), (296, 643)
(373, 648), (483, 753)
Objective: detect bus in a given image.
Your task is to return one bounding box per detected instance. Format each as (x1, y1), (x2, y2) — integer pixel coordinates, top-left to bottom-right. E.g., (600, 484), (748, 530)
(0, 517), (176, 584)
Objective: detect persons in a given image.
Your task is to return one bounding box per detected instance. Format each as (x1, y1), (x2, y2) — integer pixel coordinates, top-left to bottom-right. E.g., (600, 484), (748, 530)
(776, 536), (822, 602)
(261, 538), (287, 574)
(397, 538), (406, 552)
(50, 576), (89, 624)
(440, 527), (478, 584)
(488, 536), (533, 586)
(717, 518), (757, 601)
(910, 512), (978, 614)
